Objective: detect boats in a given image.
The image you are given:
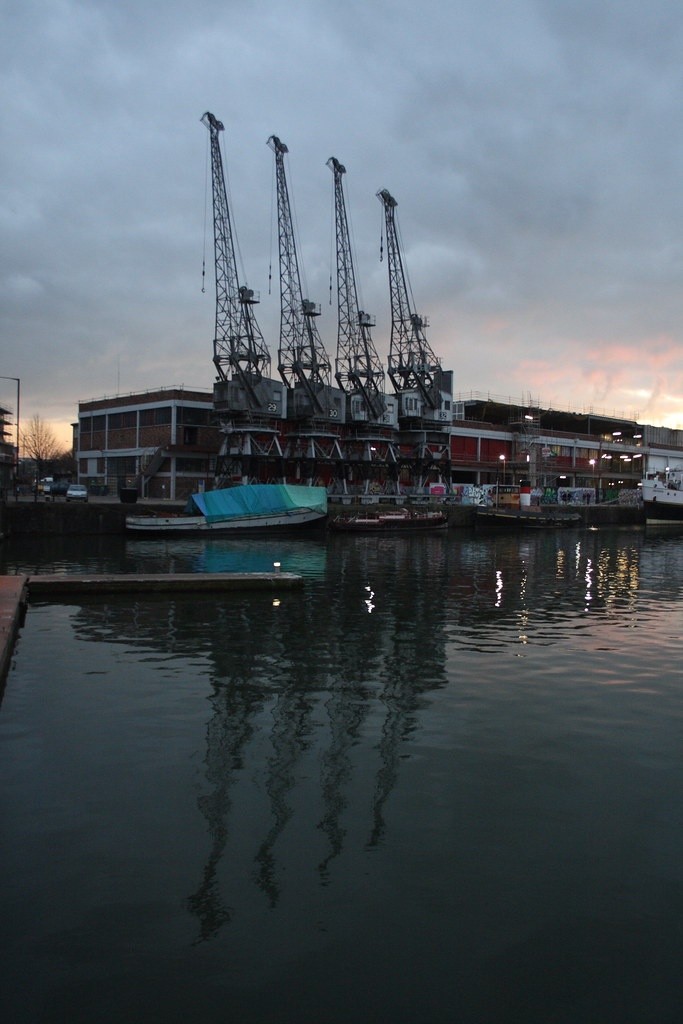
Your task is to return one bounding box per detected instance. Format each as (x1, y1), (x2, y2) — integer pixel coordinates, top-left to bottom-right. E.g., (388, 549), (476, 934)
(474, 506), (583, 529)
(332, 507), (449, 532)
(124, 484), (329, 537)
(641, 464), (683, 526)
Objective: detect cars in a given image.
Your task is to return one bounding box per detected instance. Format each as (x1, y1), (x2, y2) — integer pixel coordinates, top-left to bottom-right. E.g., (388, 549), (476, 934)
(66, 484), (90, 503)
(43, 480), (70, 497)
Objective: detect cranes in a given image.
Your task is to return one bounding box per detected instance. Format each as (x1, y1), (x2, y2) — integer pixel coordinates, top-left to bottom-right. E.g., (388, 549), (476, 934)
(197, 110), (455, 499)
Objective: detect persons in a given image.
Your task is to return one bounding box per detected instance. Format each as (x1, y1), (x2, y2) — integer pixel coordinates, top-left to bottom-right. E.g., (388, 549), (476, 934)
(561, 491), (572, 506)
(582, 491), (591, 505)
(602, 488), (606, 502)
(596, 488), (600, 503)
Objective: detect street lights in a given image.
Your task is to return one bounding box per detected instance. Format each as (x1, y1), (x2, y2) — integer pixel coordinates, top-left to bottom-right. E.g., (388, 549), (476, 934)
(589, 459), (596, 488)
(499, 454), (506, 484)
